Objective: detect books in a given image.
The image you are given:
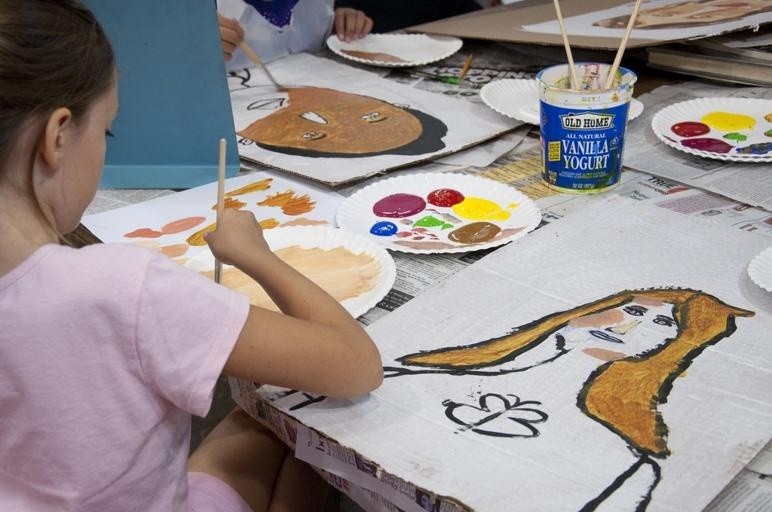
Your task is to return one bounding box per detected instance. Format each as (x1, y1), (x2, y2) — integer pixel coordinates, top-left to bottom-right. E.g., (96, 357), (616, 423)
(643, 33), (772, 88)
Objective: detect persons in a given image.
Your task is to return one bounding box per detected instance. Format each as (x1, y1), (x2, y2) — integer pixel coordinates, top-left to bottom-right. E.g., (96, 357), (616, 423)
(0, 0), (385, 512)
(215, 0), (375, 75)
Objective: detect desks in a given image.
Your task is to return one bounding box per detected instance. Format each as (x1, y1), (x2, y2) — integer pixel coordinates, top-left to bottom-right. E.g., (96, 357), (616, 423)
(69, 46), (772, 511)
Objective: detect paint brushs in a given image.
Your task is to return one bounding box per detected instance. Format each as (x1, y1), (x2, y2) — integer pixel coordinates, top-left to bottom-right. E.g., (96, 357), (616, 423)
(240, 43), (283, 89)
(215, 139), (227, 283)
(554, 0), (642, 92)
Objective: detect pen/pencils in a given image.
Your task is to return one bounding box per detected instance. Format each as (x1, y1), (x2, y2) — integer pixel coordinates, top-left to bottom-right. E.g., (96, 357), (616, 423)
(460, 53), (474, 80)
(405, 69), (478, 88)
